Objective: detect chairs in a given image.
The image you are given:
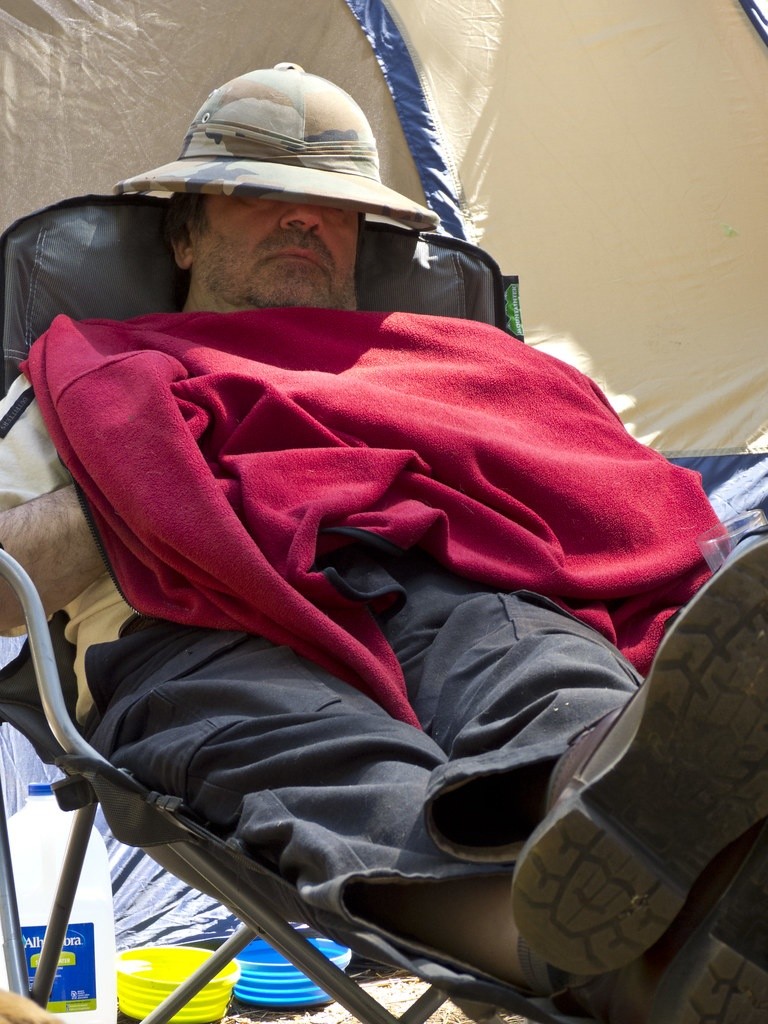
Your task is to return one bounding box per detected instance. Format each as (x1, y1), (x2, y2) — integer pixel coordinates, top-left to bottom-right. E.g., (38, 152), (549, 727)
(0, 194), (768, 1023)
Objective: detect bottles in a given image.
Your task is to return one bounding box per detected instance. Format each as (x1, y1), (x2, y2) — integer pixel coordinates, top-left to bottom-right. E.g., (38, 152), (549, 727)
(6, 784), (118, 1024)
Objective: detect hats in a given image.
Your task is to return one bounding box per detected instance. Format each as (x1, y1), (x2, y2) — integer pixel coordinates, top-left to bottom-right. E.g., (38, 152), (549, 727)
(112, 62), (442, 230)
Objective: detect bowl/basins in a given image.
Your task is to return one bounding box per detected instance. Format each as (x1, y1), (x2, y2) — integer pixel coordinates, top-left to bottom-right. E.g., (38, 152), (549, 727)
(233, 937), (351, 1006)
(117, 945), (239, 1023)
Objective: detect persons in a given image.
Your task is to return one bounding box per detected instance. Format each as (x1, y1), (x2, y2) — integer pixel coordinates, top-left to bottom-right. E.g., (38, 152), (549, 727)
(0, 58), (768, 1024)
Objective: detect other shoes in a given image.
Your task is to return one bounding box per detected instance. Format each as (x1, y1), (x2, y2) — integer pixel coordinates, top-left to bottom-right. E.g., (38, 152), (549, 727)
(506, 526), (768, 979)
(587, 801), (768, 1024)
